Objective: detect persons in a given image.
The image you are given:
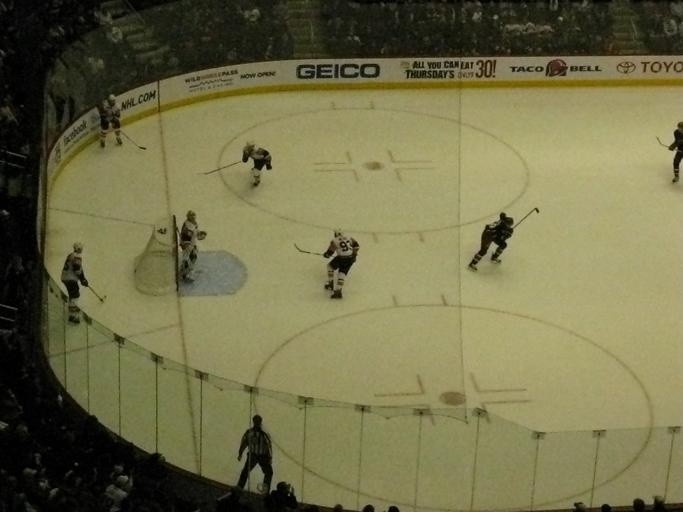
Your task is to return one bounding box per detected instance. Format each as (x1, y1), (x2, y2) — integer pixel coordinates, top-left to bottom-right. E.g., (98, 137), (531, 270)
(668, 122), (683, 183)
(95, 93), (121, 147)
(215, 487), (249, 509)
(237, 414), (274, 496)
(322, 227), (359, 297)
(240, 139), (271, 186)
(177, 210), (208, 281)
(467, 211), (514, 272)
(0, 313), (163, 512)
(264, 481), (298, 509)
(44, 1), (681, 136)
(59, 241), (90, 321)
